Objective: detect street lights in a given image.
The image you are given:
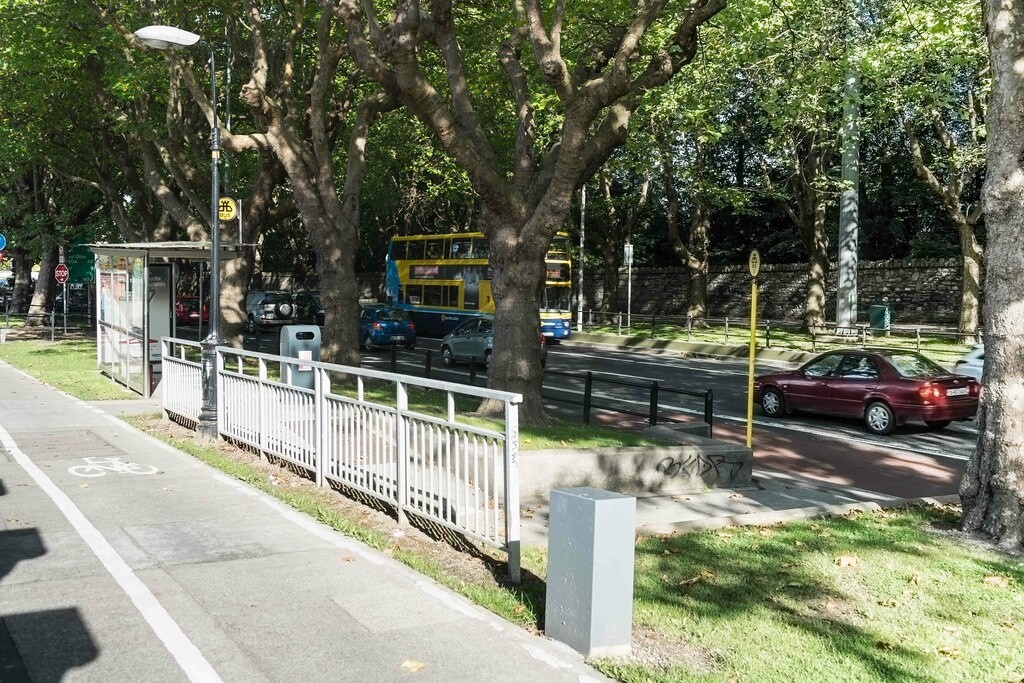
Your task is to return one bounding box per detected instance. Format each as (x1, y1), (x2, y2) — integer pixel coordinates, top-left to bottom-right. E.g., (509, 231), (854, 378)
(134, 24), (223, 452)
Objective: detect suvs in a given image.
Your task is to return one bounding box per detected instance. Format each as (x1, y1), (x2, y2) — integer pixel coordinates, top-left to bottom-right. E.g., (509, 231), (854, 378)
(246, 291), (299, 334)
(291, 290), (362, 328)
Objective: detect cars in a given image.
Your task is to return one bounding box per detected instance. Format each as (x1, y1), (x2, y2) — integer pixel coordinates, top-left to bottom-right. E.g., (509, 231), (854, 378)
(169, 295), (208, 324)
(54, 288), (96, 315)
(753, 349), (982, 435)
(356, 303), (417, 352)
(440, 315), (548, 379)
(954, 349), (985, 390)
(0, 278), (36, 311)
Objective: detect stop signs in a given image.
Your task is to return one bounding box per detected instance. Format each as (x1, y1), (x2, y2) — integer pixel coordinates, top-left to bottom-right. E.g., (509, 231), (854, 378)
(55, 264), (70, 283)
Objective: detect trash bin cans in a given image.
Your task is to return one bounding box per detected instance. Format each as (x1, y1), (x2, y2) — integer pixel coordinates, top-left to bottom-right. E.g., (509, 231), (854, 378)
(279, 324), (322, 405)
(869, 304), (895, 338)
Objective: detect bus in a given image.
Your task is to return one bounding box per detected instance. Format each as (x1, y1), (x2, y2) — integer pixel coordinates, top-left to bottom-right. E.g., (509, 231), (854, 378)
(386, 231), (571, 344)
